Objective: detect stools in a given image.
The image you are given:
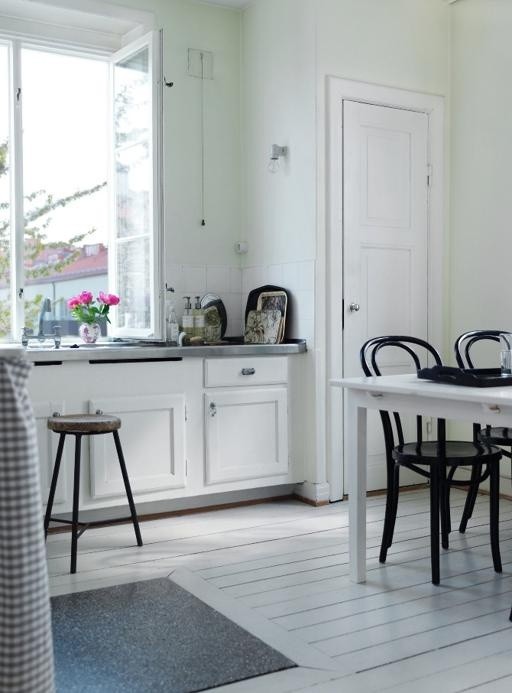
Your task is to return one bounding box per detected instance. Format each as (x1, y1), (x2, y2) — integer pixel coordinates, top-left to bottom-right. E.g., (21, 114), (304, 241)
(43, 413), (143, 575)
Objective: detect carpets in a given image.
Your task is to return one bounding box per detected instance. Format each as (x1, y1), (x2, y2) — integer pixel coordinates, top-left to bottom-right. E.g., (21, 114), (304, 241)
(50, 576), (298, 692)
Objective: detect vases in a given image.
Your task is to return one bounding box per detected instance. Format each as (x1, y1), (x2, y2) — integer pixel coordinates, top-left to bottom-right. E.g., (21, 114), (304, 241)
(77, 322), (100, 343)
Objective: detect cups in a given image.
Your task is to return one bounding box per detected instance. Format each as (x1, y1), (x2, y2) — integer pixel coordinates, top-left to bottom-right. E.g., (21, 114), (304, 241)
(499, 333), (511, 377)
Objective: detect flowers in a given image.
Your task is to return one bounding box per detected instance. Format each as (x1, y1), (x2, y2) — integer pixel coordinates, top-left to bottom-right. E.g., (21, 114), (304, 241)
(66, 290), (120, 324)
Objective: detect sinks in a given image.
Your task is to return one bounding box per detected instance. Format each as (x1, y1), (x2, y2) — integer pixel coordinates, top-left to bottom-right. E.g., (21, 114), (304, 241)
(27, 342), (142, 349)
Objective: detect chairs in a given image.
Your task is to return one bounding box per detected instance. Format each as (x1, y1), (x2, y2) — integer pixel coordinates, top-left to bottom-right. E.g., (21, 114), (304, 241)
(358, 329), (512, 586)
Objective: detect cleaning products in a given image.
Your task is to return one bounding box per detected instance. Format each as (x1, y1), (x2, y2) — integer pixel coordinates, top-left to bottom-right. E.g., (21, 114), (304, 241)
(193, 296), (206, 337)
(168, 307), (179, 344)
(182, 296), (194, 338)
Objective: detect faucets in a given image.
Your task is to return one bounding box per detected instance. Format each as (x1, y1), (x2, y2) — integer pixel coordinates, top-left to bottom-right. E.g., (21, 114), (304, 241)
(39, 298), (52, 336)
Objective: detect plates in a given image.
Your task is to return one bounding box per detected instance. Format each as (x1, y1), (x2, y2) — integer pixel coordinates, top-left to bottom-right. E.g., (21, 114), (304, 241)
(245, 291), (287, 345)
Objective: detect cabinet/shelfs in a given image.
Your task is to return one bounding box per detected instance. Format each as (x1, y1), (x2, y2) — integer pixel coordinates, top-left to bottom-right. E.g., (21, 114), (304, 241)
(195, 356), (302, 512)
(23, 355), (80, 529)
(81, 356), (196, 523)
